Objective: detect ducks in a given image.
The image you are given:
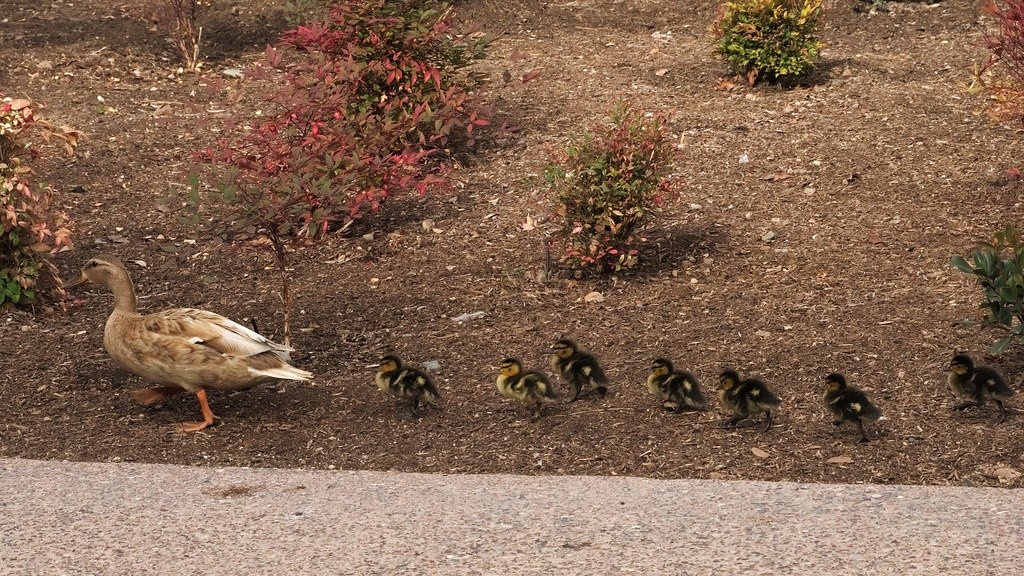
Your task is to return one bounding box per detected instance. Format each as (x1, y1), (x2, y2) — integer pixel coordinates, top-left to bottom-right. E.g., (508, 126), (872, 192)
(60, 255), (315, 433)
(818, 373), (882, 443)
(492, 358), (559, 418)
(707, 369), (782, 433)
(364, 354), (442, 417)
(941, 355), (1013, 424)
(541, 339), (609, 404)
(642, 357), (707, 414)
